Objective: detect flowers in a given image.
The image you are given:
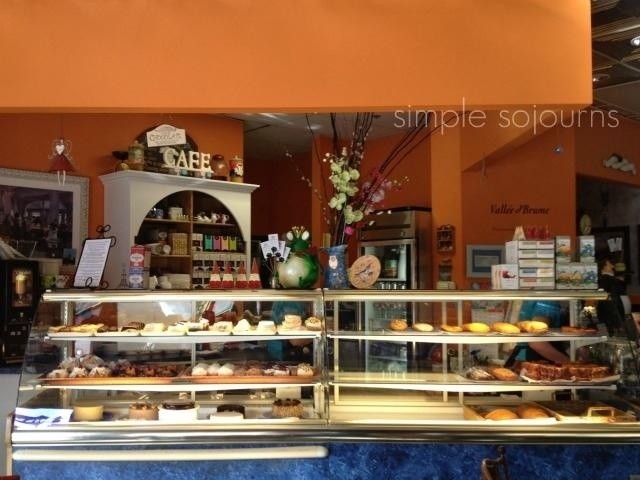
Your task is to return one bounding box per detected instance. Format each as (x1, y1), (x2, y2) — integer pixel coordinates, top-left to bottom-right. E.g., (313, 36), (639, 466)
(284, 109), (479, 246)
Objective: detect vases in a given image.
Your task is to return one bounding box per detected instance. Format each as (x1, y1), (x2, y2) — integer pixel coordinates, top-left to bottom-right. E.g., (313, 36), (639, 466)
(322, 243), (351, 290)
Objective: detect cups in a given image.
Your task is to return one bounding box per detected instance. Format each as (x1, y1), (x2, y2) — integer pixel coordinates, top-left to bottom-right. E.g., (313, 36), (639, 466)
(55, 274), (69, 288)
(228, 155), (244, 183)
(153, 208), (163, 219)
(41, 275), (57, 289)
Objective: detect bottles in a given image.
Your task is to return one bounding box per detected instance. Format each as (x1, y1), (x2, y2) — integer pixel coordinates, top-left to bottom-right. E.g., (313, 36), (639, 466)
(383, 249), (399, 278)
(399, 344), (407, 360)
(177, 213), (189, 220)
(525, 224), (551, 240)
(128, 139), (145, 171)
(210, 154), (228, 181)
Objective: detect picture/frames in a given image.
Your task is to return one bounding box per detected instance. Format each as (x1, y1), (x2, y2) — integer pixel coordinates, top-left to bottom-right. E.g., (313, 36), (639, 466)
(0, 167), (90, 276)
(466, 244), (507, 279)
(587, 224), (634, 286)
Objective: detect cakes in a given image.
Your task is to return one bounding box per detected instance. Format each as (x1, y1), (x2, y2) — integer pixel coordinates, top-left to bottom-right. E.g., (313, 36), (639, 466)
(46, 315), (323, 422)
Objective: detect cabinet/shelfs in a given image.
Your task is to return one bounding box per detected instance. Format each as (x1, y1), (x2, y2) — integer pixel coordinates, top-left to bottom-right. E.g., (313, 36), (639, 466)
(327, 292), (640, 427)
(99, 170), (260, 288)
(14, 287), (327, 437)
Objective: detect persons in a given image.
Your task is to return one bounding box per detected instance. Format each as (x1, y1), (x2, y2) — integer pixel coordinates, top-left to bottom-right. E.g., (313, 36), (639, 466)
(47, 138), (75, 186)
(514, 300), (590, 364)
(597, 257), (627, 336)
(272, 302), (313, 361)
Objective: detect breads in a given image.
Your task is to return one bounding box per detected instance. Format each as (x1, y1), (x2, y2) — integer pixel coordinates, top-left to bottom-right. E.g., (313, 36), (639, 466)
(485, 403), (549, 420)
(387, 320), (598, 335)
(466, 361), (610, 381)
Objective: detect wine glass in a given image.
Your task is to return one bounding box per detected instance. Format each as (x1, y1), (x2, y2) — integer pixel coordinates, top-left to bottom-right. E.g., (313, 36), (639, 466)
(112, 150), (134, 168)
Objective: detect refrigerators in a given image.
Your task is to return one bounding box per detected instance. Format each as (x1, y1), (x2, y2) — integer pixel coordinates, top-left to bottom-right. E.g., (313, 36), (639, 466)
(354, 209), (433, 385)
